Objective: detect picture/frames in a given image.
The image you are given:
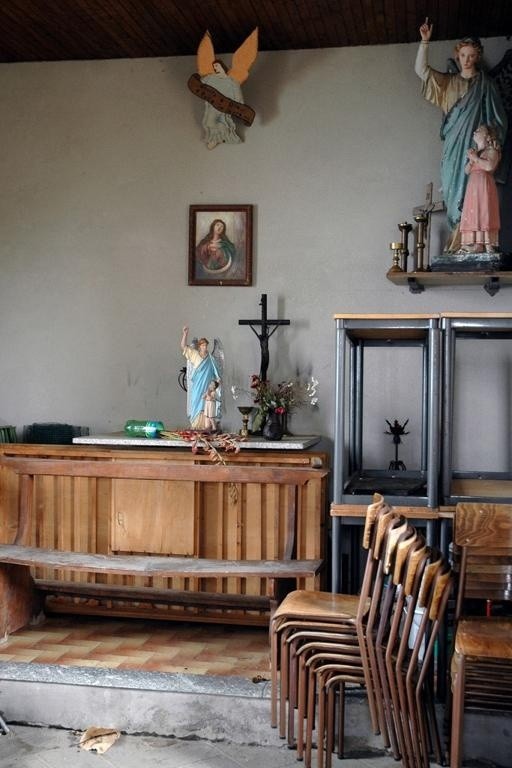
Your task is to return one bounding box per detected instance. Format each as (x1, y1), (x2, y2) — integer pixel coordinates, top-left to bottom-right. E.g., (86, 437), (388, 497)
(189, 203), (253, 285)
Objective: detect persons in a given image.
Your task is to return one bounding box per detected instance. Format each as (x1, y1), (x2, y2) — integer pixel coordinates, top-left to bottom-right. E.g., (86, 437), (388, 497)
(194, 219), (237, 280)
(177, 322), (225, 433)
(456, 113), (502, 255)
(414, 11), (509, 257)
(199, 380), (220, 434)
(194, 59), (243, 154)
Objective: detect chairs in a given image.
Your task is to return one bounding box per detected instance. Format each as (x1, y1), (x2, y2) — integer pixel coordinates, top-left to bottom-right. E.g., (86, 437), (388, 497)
(268, 498), (512, 768)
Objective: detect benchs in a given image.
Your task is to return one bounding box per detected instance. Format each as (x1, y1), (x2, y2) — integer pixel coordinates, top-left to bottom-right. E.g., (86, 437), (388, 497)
(0, 542), (323, 642)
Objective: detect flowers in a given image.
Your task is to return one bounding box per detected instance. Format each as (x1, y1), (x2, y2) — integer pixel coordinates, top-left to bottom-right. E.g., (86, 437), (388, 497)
(230, 374), (320, 431)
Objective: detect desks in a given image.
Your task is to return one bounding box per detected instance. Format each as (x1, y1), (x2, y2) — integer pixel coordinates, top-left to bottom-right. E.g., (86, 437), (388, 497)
(331, 311), (512, 595)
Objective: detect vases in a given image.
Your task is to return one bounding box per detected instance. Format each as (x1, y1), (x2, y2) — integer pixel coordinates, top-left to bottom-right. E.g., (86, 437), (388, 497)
(263, 409), (284, 442)
